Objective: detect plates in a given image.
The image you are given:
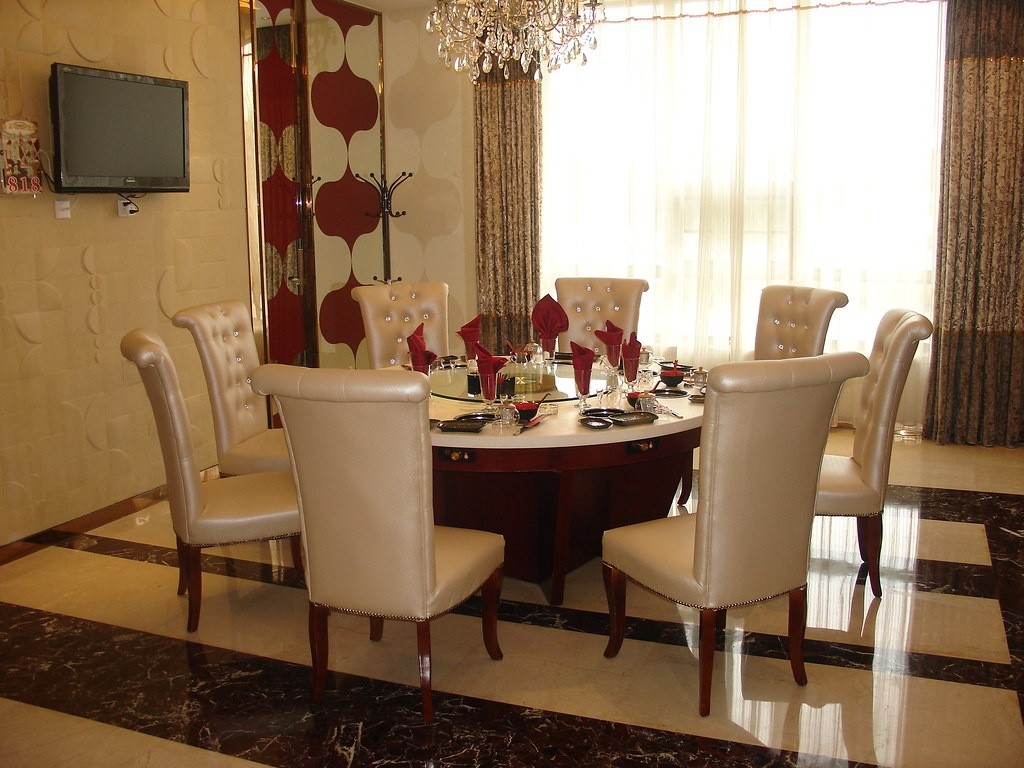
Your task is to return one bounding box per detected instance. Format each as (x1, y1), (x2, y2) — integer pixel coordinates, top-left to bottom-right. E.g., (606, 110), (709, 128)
(429, 412), (501, 433)
(578, 407), (659, 429)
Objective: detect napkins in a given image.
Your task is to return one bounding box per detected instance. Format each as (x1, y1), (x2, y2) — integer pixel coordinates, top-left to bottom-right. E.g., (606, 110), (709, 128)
(407, 323), (437, 376)
(622, 332), (642, 382)
(532, 294), (569, 352)
(570, 341), (595, 395)
(474, 342), (508, 400)
(454, 316), (482, 360)
(594, 320), (623, 367)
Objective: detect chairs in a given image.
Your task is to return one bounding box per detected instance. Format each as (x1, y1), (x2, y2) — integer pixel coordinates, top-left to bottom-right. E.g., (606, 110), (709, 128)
(171, 301), (292, 478)
(556, 277), (649, 358)
(251, 364), (504, 724)
(815, 308), (932, 597)
(603, 351), (870, 717)
(120, 327), (300, 632)
(351, 283), (449, 370)
(678, 285), (849, 510)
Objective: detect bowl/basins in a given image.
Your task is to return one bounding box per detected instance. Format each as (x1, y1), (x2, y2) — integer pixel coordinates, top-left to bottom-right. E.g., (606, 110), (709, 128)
(513, 401), (539, 423)
(660, 371), (684, 388)
(627, 392), (643, 410)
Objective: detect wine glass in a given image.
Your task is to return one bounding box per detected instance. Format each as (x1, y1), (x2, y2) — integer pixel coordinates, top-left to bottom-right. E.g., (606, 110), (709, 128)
(605, 344), (621, 391)
(478, 374), (498, 413)
(622, 357), (639, 394)
(573, 369), (592, 409)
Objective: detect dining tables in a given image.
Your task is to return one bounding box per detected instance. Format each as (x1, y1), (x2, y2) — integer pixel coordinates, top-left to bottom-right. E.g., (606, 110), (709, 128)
(374, 356), (709, 604)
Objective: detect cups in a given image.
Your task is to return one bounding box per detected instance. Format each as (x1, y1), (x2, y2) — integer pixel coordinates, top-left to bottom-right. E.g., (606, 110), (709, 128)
(409, 365), (430, 377)
(693, 366), (708, 389)
(638, 390), (655, 414)
(541, 337), (556, 362)
(497, 398), (520, 430)
(464, 341), (479, 360)
(639, 347), (652, 364)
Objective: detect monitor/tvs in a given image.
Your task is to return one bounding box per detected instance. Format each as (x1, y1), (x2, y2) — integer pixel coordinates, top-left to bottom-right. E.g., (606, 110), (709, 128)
(48, 62), (189, 194)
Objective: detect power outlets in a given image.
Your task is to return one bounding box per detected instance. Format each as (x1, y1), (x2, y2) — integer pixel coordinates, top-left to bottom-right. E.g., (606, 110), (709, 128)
(55, 200), (71, 218)
(117, 201), (133, 216)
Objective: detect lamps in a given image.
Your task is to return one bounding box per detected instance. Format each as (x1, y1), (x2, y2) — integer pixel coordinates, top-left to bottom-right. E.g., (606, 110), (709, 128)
(425, 0), (605, 85)
